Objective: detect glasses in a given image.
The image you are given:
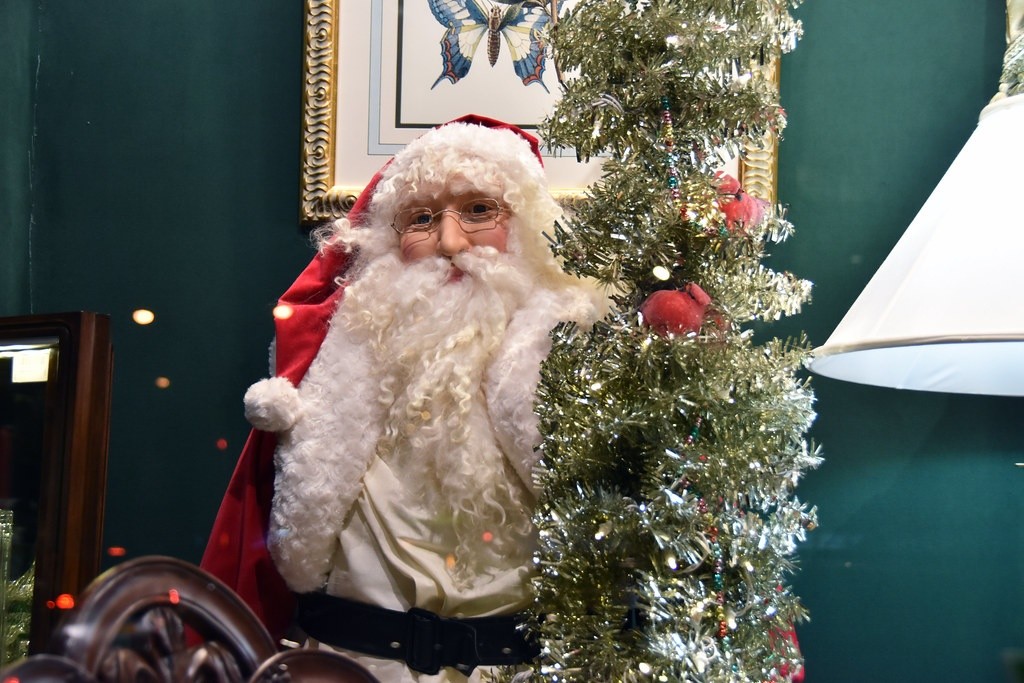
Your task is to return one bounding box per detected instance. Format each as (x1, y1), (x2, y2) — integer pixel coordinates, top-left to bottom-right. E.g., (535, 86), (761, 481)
(390, 198), (512, 234)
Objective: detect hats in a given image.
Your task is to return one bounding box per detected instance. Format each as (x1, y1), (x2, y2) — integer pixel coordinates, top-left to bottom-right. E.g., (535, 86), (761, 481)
(244, 113), (566, 434)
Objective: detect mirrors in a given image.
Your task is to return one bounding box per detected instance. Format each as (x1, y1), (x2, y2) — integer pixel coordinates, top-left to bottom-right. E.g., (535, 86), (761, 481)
(0, 310), (115, 666)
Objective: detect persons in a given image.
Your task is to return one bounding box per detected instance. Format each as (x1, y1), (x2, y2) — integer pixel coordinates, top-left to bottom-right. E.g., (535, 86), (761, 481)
(183, 115), (618, 683)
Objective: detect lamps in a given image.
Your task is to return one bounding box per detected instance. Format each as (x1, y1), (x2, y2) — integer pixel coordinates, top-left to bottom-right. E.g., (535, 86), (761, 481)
(799, 0), (1024, 397)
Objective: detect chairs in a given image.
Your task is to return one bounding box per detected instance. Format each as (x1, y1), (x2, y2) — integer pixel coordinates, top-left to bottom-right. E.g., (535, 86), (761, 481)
(0, 554), (387, 683)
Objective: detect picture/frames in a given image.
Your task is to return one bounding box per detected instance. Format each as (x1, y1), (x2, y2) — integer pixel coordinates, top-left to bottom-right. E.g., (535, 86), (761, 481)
(299, 0), (777, 237)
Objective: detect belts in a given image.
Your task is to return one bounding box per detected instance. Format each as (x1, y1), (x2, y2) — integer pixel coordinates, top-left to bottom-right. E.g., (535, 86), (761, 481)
(295, 590), (543, 674)
